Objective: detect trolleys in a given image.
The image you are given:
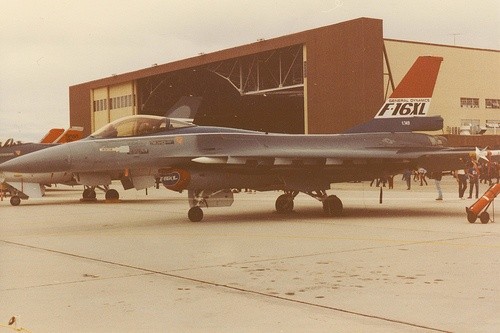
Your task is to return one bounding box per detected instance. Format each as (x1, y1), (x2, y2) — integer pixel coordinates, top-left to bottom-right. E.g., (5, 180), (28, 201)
(465, 189), (500, 225)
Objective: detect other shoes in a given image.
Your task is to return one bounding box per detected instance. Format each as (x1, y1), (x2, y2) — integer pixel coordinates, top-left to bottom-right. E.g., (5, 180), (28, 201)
(461, 197), (466, 200)
(475, 196), (478, 198)
(435, 198), (442, 200)
(370, 183), (431, 189)
(468, 197), (472, 199)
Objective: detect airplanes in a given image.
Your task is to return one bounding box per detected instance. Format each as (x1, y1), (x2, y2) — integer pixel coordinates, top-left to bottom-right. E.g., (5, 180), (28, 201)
(0, 54), (500, 223)
(0, 126), (84, 206)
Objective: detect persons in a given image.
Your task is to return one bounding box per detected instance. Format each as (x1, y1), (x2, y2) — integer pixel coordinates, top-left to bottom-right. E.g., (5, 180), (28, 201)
(369, 166), (428, 190)
(432, 167), (443, 200)
(471, 160), (500, 186)
(454, 157), (467, 200)
(468, 162), (479, 199)
